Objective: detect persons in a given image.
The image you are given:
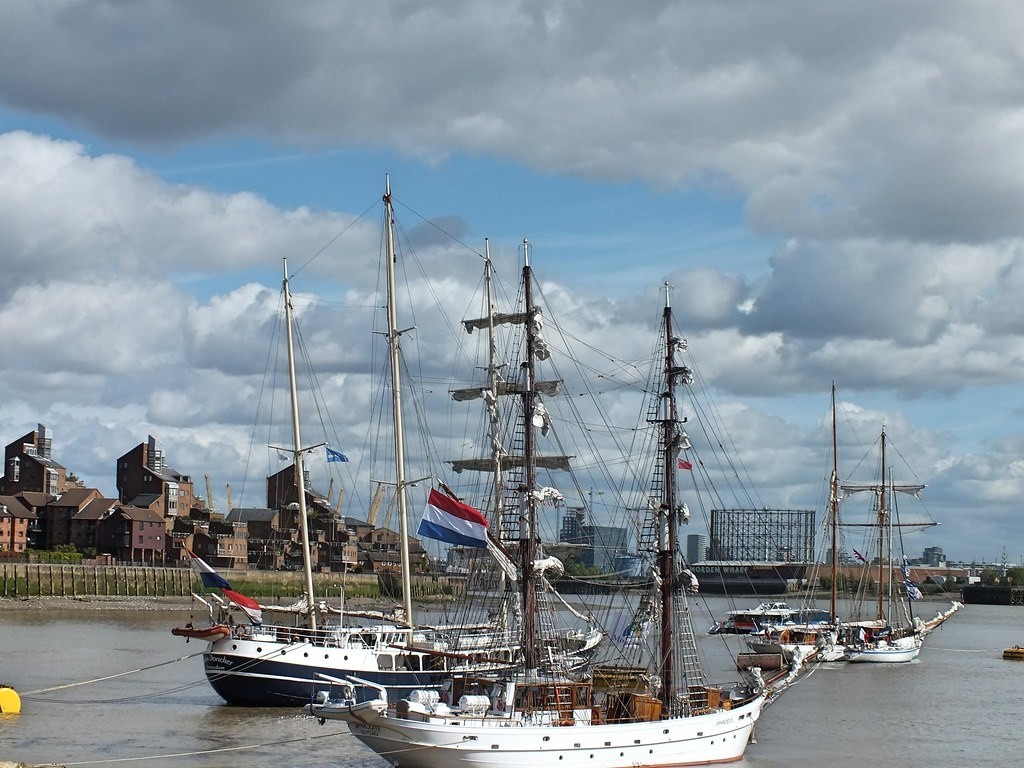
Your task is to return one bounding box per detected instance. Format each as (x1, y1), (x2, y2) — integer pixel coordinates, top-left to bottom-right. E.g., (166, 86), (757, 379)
(835, 614), (839, 624)
(863, 630), (870, 645)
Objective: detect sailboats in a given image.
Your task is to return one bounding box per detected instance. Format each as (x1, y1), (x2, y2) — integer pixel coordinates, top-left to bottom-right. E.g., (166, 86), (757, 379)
(166, 174), (968, 768)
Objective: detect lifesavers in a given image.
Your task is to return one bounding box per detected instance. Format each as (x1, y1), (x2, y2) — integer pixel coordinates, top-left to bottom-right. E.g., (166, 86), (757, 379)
(235, 624), (248, 638)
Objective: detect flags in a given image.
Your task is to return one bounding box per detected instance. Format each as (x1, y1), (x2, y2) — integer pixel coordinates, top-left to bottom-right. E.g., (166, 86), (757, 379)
(326, 447), (349, 462)
(856, 625), (873, 643)
(677, 458), (693, 470)
(412, 486), (489, 550)
(185, 546), (263, 625)
(904, 579), (923, 602)
